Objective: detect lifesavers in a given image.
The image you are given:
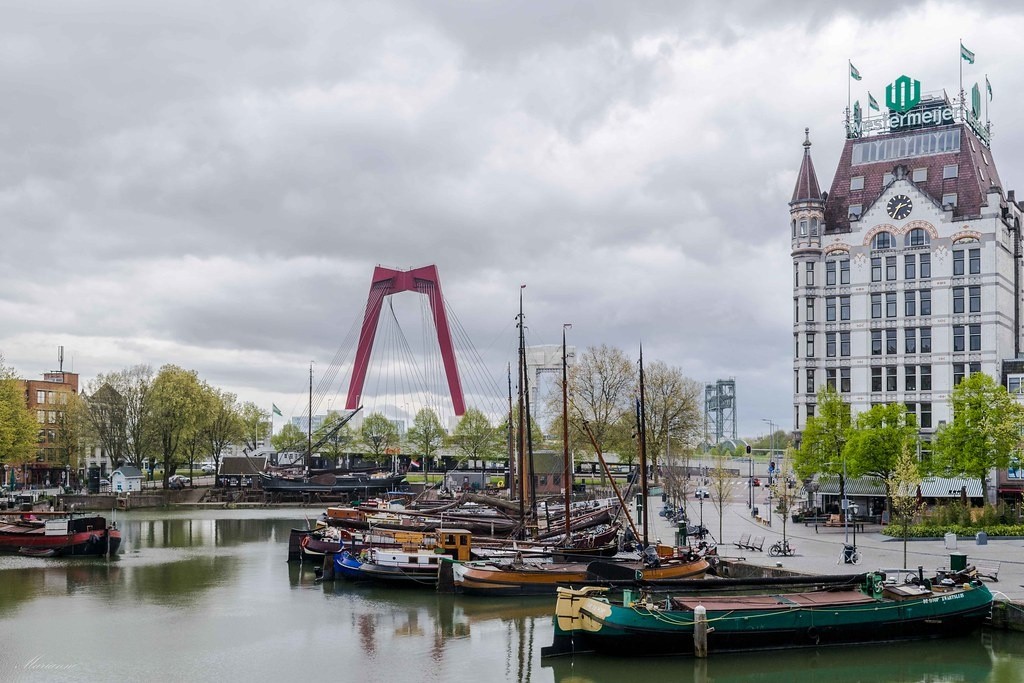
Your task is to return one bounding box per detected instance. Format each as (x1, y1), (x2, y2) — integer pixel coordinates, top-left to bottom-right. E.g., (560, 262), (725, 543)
(302, 538), (308, 546)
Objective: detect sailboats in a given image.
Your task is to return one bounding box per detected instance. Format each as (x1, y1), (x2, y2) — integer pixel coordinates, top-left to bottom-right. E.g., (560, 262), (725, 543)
(289, 282), (712, 594)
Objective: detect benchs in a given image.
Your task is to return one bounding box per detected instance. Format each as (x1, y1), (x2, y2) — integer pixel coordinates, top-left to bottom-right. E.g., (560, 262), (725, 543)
(756, 515), (762, 523)
(816, 522), (870, 533)
(763, 520), (770, 526)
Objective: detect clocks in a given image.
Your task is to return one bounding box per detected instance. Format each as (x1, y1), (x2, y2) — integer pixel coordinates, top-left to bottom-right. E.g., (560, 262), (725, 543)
(886, 194), (912, 220)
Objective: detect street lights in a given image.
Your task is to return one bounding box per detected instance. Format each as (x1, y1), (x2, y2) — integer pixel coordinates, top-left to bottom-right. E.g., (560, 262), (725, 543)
(2, 464), (10, 488)
(65, 464), (72, 491)
(848, 501), (859, 557)
(761, 418), (778, 527)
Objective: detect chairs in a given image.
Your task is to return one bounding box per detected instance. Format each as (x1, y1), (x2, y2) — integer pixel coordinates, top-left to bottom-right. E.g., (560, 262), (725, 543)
(882, 511), (888, 525)
(742, 536), (765, 552)
(734, 534), (751, 548)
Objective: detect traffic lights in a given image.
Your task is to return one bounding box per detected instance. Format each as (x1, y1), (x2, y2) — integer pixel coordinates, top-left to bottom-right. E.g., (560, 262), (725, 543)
(746, 446), (751, 454)
(769, 462), (775, 472)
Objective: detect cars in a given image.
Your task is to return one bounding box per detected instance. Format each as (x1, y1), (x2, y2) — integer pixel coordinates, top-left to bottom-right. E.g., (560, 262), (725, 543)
(169, 474), (190, 484)
(748, 478), (760, 486)
(201, 465), (216, 472)
(695, 490), (710, 499)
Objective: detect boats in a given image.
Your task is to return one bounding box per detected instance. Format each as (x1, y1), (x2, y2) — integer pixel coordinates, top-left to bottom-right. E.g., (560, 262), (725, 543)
(552, 568), (996, 653)
(0, 512), (122, 559)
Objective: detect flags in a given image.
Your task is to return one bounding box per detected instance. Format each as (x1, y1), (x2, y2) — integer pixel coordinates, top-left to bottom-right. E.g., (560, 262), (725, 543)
(986, 78), (992, 101)
(961, 44), (974, 64)
(850, 63), (862, 80)
(272, 403), (282, 416)
(868, 91), (879, 111)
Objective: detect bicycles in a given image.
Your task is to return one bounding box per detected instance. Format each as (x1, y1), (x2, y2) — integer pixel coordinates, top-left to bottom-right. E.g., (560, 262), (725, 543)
(768, 537), (795, 557)
(838, 542), (865, 564)
